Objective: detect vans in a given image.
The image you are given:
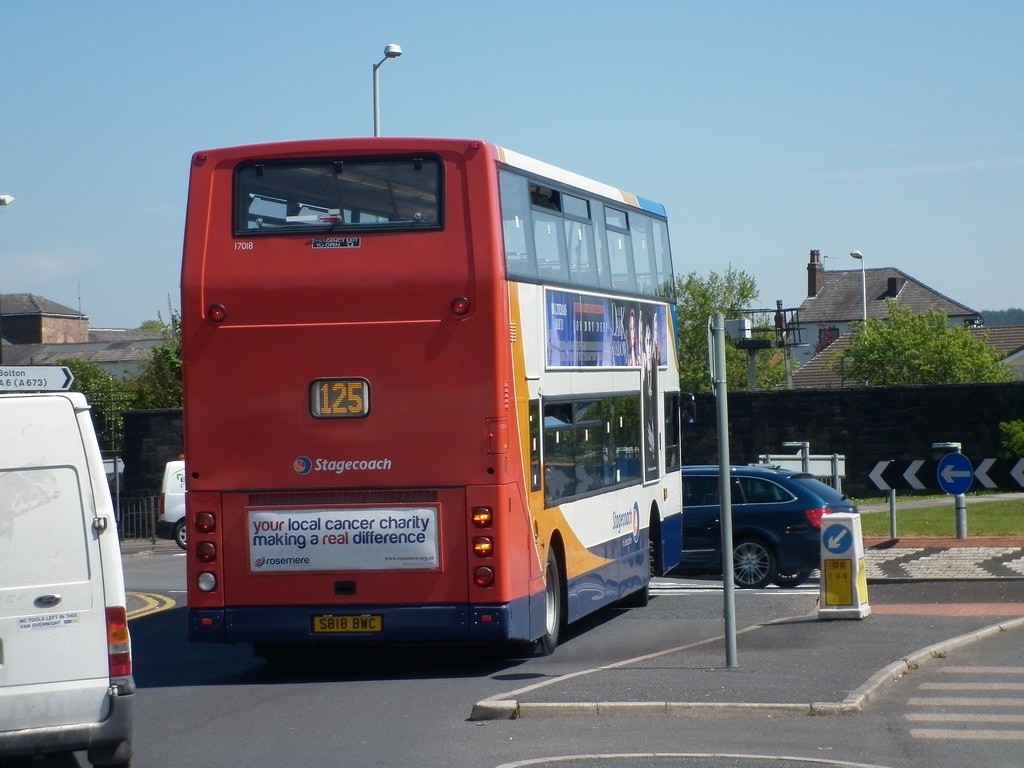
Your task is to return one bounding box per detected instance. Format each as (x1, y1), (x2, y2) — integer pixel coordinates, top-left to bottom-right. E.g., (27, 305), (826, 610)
(1, 393), (137, 768)
(159, 462), (187, 551)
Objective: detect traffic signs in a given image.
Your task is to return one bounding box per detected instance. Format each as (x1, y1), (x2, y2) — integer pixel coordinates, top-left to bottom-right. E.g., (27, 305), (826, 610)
(0, 366), (74, 390)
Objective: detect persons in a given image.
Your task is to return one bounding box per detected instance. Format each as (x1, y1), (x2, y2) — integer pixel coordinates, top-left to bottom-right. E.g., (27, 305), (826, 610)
(627, 309), (661, 366)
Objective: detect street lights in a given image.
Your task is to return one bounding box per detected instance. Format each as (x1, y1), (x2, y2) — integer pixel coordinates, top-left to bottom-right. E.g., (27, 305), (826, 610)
(850, 251), (869, 386)
(372, 43), (403, 138)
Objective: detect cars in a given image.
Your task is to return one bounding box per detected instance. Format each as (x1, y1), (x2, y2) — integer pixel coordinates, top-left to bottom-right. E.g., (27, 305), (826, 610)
(679, 464), (859, 591)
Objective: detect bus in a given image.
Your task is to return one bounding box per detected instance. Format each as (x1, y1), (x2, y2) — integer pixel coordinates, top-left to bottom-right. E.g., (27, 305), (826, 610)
(181, 138), (697, 660)
